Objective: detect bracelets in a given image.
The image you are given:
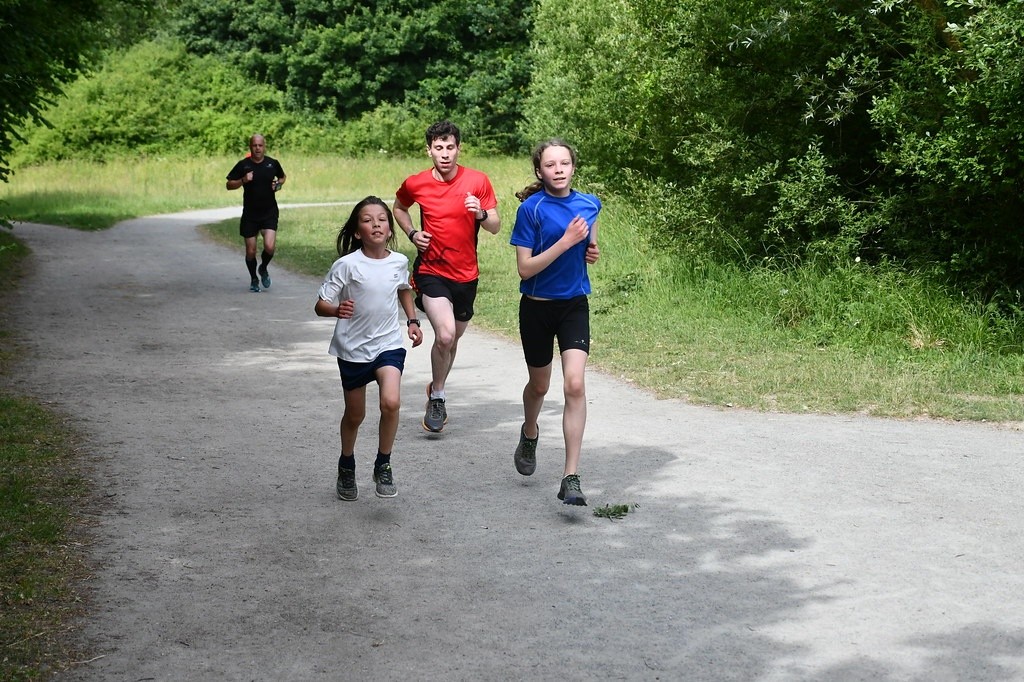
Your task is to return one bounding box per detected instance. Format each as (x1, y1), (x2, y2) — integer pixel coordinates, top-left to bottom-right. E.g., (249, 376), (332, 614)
(409, 230), (418, 242)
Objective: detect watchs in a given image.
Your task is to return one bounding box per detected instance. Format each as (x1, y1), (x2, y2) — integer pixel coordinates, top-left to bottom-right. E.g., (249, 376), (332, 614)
(475, 208), (488, 222)
(406, 319), (421, 327)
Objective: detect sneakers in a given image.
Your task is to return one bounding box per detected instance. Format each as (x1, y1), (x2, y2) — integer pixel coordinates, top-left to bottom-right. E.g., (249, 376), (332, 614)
(336, 459), (358, 501)
(557, 474), (588, 506)
(258, 264), (270, 288)
(422, 398), (444, 432)
(426, 380), (447, 423)
(250, 276), (260, 293)
(514, 422), (539, 475)
(373, 460), (398, 498)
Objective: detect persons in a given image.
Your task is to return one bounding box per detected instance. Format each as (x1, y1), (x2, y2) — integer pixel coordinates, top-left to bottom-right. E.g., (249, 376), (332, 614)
(314, 195), (423, 502)
(508, 141), (602, 506)
(393, 121), (501, 437)
(226, 133), (286, 293)
